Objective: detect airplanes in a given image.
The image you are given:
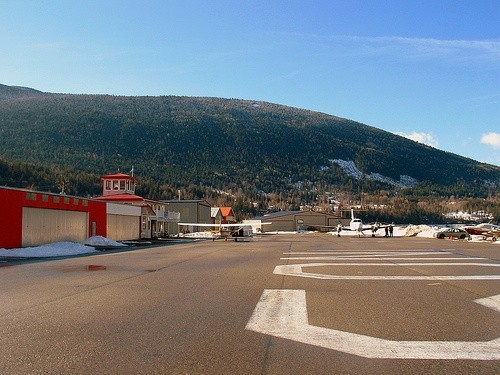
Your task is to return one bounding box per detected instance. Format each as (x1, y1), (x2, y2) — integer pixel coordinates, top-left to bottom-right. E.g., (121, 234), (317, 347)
(321, 208), (397, 238)
(464, 223), (500, 240)
(177, 216), (273, 242)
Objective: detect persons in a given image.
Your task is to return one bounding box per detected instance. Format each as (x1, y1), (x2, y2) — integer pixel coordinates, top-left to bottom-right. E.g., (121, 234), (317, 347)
(384, 226), (393, 238)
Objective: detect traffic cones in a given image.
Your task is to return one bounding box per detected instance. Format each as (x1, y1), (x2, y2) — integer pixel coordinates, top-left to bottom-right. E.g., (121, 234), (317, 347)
(451, 235), (453, 241)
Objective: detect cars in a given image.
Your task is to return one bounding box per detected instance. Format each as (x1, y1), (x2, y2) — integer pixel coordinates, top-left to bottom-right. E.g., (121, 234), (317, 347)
(307, 226), (320, 231)
(433, 228), (469, 239)
